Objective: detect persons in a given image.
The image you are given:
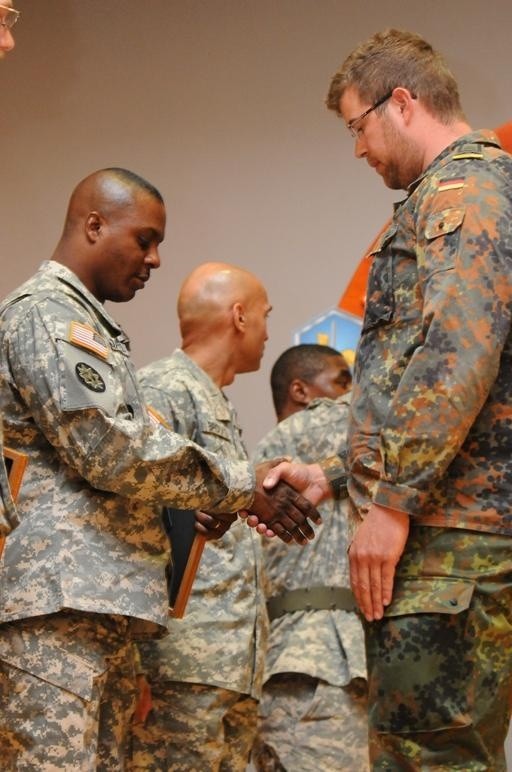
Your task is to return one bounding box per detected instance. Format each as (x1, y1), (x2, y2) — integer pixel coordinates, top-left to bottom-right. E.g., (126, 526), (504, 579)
(256, 18), (512, 772)
(0, 165), (325, 772)
(132, 255), (276, 772)
(249, 341), (371, 772)
(1, 0), (22, 545)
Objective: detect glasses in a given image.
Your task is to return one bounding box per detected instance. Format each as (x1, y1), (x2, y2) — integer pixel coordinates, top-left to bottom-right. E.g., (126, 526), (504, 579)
(346, 87), (417, 137)
